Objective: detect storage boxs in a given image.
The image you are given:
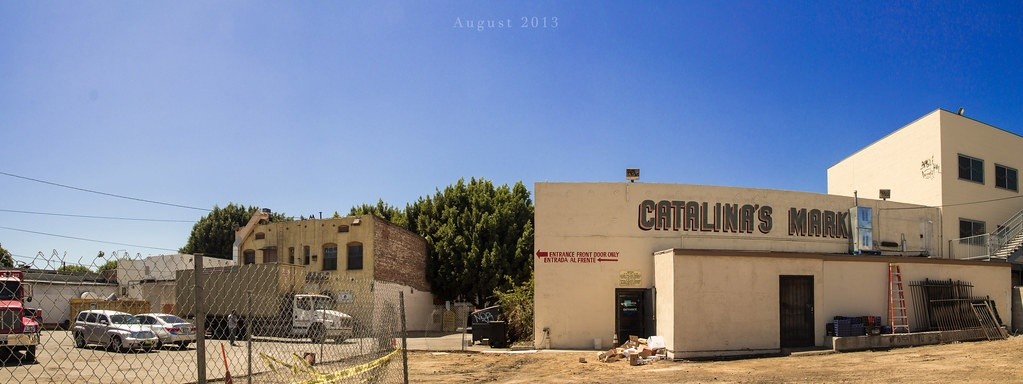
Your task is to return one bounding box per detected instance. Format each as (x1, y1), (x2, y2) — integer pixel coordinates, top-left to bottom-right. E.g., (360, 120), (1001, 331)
(579, 357), (587, 363)
(826, 315), (891, 337)
(599, 335), (667, 366)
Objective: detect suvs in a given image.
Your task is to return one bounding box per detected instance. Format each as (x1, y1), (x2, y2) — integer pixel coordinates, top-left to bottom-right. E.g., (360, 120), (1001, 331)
(72, 309), (158, 353)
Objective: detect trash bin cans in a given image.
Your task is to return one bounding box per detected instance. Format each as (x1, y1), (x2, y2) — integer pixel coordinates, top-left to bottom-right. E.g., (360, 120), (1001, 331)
(471, 305), (500, 344)
(488, 321), (508, 348)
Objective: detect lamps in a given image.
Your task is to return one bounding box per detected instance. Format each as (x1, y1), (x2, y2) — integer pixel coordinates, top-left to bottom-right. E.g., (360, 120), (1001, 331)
(958, 107), (964, 115)
(879, 189), (891, 201)
(625, 168), (640, 182)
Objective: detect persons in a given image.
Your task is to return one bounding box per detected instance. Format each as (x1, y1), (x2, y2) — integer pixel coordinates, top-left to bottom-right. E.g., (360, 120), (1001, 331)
(228, 310), (236, 346)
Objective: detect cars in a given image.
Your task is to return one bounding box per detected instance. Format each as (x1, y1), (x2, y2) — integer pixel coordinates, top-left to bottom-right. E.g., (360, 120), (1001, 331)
(127, 312), (197, 350)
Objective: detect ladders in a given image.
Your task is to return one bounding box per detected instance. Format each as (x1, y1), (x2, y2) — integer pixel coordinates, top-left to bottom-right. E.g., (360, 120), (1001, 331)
(888, 263), (909, 334)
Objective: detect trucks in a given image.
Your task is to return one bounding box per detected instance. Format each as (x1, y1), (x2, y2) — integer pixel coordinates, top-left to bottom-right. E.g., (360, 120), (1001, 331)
(175, 262), (354, 344)
(69, 297), (151, 334)
(0, 268), (44, 361)
